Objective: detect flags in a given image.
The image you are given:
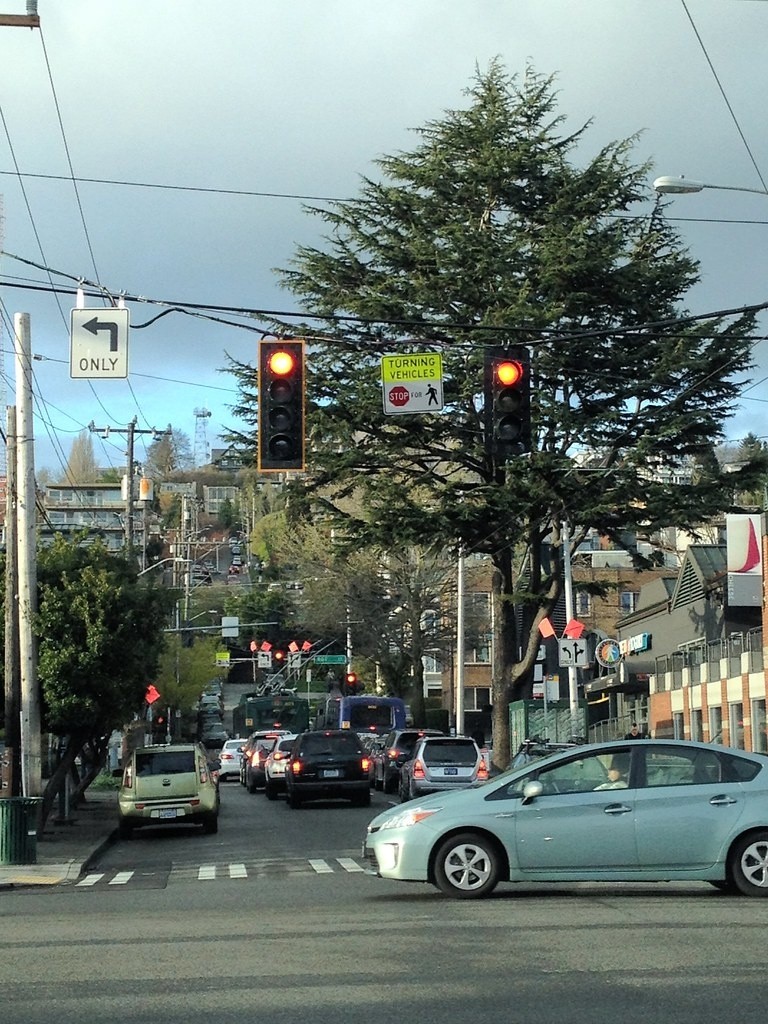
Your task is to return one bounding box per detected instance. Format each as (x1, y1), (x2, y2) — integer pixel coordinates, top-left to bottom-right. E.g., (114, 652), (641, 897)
(537, 617), (587, 641)
(144, 684), (160, 704)
(250, 640), (312, 653)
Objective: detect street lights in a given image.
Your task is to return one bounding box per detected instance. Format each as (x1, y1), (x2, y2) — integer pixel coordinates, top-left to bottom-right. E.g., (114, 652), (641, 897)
(232, 514), (248, 561)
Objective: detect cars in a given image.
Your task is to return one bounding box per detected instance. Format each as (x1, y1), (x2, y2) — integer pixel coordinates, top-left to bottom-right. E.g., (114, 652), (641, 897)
(362, 742), (768, 900)
(113, 742), (221, 840)
(196, 683), (230, 749)
(192, 562), (217, 586)
(358, 729), (489, 803)
(499, 734), (610, 797)
(217, 730), (303, 799)
(226, 538), (241, 586)
(286, 731), (372, 809)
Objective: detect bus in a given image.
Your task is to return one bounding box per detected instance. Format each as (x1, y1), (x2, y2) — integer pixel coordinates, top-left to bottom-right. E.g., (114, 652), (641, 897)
(319, 696), (406, 731)
(233, 639), (336, 738)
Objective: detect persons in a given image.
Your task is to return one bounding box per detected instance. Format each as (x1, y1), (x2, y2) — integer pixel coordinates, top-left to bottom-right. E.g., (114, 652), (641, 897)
(594, 723), (647, 790)
(327, 668), (335, 679)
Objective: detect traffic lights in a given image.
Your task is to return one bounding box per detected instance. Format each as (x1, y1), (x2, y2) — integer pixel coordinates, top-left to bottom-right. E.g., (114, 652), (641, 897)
(257, 341), (303, 472)
(153, 715), (164, 726)
(483, 346), (533, 455)
(345, 673), (358, 697)
(274, 649), (285, 668)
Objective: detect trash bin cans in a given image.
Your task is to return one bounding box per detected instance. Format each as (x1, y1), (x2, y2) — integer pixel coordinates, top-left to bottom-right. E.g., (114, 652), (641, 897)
(0, 795), (45, 866)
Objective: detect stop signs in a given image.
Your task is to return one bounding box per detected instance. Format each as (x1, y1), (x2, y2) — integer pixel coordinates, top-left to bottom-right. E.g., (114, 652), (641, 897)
(390, 387), (409, 407)
